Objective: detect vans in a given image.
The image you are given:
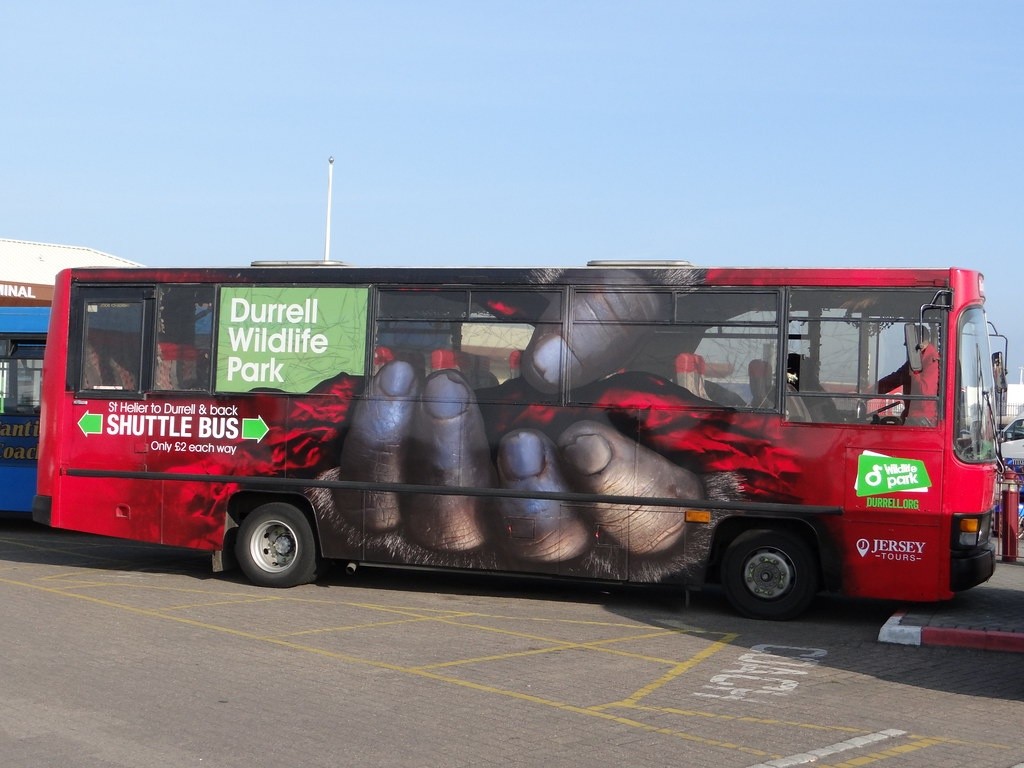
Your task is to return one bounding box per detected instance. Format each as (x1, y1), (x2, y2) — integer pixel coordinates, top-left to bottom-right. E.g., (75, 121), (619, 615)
(996, 411), (1024, 458)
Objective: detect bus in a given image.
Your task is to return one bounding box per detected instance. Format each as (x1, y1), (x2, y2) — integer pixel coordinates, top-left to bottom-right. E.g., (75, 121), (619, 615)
(37, 259), (1008, 619)
(0, 306), (215, 511)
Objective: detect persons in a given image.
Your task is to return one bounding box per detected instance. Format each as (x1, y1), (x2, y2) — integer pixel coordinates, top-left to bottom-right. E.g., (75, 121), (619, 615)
(870, 325), (940, 423)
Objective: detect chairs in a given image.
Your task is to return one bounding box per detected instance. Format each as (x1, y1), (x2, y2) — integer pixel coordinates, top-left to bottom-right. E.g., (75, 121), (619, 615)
(0, 391), (36, 413)
(84, 333), (209, 393)
(369, 344), (838, 424)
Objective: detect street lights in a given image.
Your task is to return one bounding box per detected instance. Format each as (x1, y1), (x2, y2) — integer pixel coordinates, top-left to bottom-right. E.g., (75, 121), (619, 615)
(325, 156), (335, 260)
